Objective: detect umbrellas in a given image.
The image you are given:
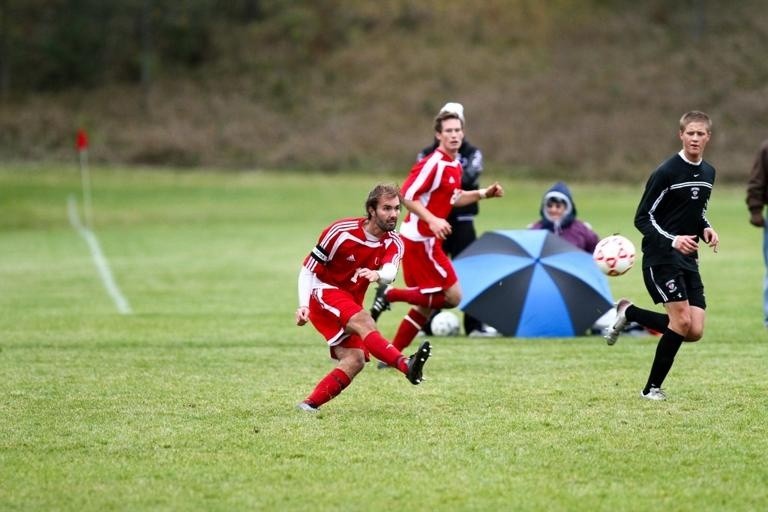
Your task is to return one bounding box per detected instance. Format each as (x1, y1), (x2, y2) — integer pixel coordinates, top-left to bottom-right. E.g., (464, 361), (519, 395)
(450, 225), (614, 339)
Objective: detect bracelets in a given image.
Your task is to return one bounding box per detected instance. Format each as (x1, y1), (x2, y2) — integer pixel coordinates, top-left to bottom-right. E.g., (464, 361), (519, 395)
(479, 189), (487, 200)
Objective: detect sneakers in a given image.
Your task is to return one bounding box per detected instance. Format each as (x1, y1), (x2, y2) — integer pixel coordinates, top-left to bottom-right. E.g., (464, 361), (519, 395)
(403, 341), (433, 385)
(371, 283), (392, 322)
(604, 298), (633, 345)
(297, 402), (318, 413)
(639, 388), (666, 401)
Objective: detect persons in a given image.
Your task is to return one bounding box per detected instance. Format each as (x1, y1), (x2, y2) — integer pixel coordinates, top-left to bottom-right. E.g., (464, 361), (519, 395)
(527, 180), (603, 336)
(367, 110), (505, 369)
(414, 100), (488, 338)
(602, 110), (721, 401)
(293, 181), (432, 412)
(745, 139), (768, 329)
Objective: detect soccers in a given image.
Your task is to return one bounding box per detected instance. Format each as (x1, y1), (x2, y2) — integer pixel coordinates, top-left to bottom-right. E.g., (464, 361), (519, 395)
(594, 235), (635, 275)
(432, 311), (461, 336)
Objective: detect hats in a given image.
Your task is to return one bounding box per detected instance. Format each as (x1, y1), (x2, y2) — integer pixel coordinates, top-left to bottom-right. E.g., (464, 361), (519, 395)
(439, 103), (464, 125)
